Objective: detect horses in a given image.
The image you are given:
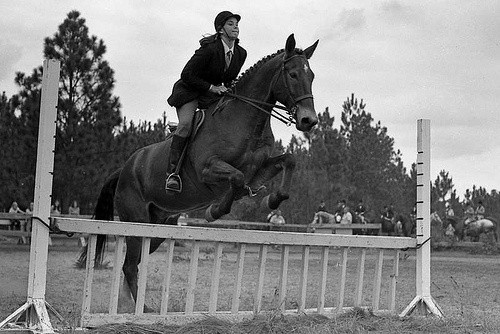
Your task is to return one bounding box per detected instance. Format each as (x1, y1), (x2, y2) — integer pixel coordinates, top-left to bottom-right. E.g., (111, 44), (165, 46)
(310, 212), (498, 244)
(75, 33), (319, 315)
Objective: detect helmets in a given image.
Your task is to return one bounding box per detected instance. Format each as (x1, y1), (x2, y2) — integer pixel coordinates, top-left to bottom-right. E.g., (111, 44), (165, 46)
(214, 11), (241, 32)
(321, 200), (325, 203)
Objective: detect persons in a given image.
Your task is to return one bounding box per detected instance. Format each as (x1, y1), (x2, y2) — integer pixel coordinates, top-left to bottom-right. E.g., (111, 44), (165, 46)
(318, 199), (416, 235)
(177, 212), (188, 226)
(431, 201), (484, 236)
(267, 209), (285, 249)
(165, 11), (247, 191)
(68, 201), (80, 215)
(51, 199), (61, 214)
(9, 202), (34, 231)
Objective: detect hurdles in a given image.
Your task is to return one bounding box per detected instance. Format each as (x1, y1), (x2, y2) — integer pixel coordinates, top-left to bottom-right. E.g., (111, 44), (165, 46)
(0, 57), (449, 334)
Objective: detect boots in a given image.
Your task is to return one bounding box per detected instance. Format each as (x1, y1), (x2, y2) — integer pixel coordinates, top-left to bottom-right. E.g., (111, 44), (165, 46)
(165, 133), (191, 195)
